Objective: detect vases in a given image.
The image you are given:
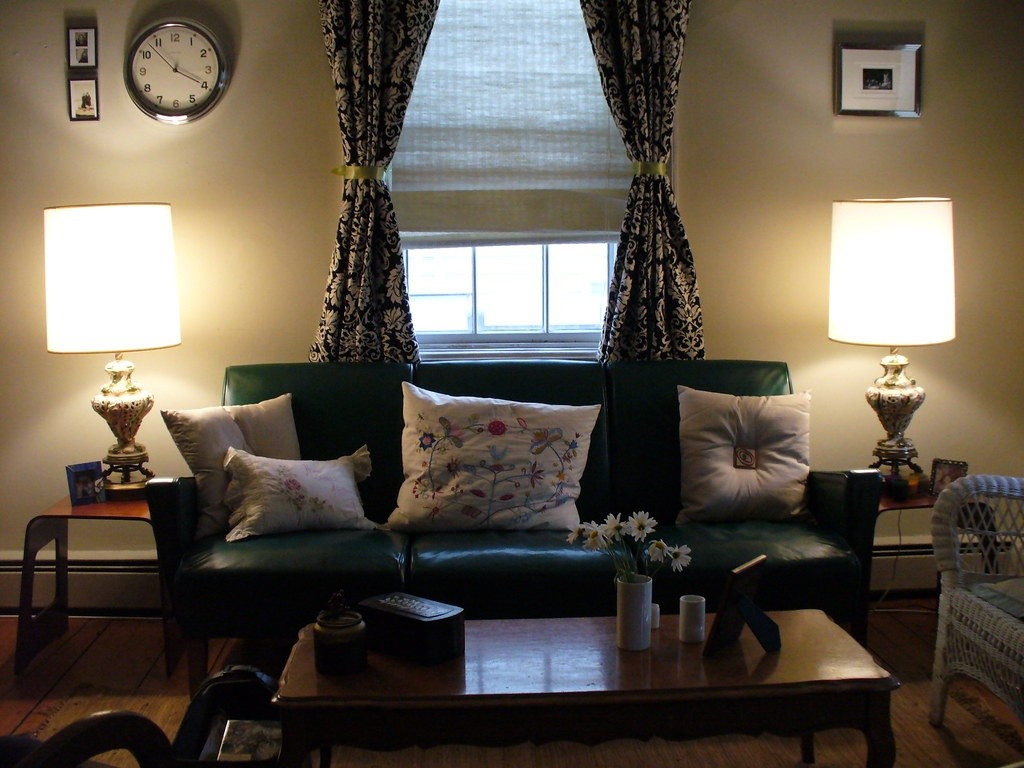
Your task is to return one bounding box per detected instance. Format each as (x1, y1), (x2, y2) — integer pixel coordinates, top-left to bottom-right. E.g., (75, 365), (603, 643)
(617, 575), (653, 651)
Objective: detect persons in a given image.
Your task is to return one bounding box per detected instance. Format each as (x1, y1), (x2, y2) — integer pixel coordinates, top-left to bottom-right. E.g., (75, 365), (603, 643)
(225, 722), (282, 760)
(76, 479), (93, 496)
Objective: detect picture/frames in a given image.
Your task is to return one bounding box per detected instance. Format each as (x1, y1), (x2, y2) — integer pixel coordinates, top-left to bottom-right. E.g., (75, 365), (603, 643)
(833, 42), (920, 118)
(68, 71), (100, 121)
(67, 25), (98, 69)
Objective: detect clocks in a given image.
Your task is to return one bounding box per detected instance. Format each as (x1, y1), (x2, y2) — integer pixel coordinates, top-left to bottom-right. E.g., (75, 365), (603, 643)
(123, 17), (231, 126)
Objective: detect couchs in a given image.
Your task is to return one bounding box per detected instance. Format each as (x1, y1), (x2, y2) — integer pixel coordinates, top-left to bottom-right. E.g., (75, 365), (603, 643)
(145, 357), (884, 702)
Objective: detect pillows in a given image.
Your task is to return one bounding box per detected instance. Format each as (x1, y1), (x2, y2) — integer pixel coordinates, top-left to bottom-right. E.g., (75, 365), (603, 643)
(676, 385), (812, 525)
(220, 443), (381, 541)
(383, 381), (601, 533)
(161, 392), (301, 537)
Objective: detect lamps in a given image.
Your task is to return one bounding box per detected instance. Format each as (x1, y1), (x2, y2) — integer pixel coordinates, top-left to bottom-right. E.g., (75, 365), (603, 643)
(43, 203), (180, 482)
(826, 197), (957, 476)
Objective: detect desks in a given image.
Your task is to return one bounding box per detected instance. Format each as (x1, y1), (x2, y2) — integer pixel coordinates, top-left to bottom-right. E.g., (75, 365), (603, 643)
(868, 496), (998, 614)
(265, 611), (900, 768)
(13, 496), (179, 678)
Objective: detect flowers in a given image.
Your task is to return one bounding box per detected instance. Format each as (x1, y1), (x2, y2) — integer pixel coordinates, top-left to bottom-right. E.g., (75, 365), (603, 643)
(564, 511), (691, 582)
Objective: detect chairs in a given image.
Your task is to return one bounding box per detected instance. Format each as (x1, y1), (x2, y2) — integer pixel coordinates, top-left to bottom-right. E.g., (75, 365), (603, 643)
(925, 476), (1024, 732)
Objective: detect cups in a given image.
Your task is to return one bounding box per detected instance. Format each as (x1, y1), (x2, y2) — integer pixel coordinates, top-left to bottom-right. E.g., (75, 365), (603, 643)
(312, 610), (368, 677)
(679, 595), (706, 643)
